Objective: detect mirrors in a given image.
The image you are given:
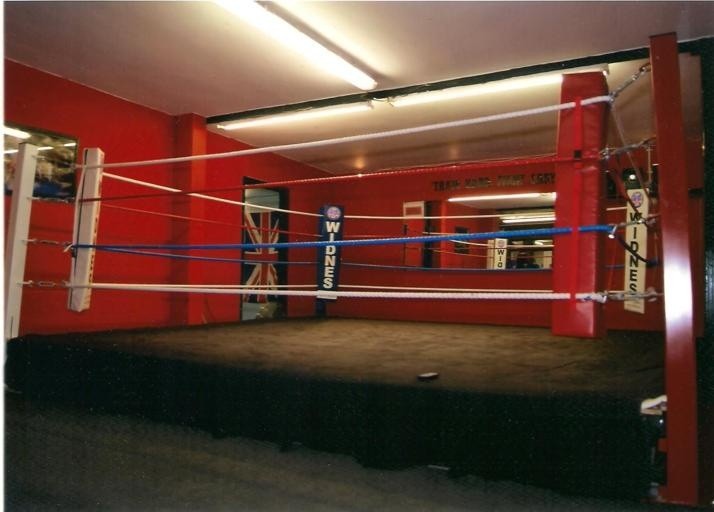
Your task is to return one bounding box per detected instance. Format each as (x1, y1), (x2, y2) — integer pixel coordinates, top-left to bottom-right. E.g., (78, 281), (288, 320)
(441, 191), (557, 271)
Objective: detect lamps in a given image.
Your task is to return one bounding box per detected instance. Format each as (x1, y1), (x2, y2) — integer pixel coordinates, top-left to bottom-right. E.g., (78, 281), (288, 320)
(218, 93), (374, 130)
(389, 61), (610, 109)
(203, 0), (379, 92)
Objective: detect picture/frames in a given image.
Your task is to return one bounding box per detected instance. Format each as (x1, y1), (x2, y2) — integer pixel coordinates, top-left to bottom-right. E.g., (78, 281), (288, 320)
(3, 121), (79, 205)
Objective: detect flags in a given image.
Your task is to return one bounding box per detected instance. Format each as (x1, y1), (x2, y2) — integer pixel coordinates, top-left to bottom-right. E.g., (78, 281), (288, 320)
(241, 210), (280, 304)
